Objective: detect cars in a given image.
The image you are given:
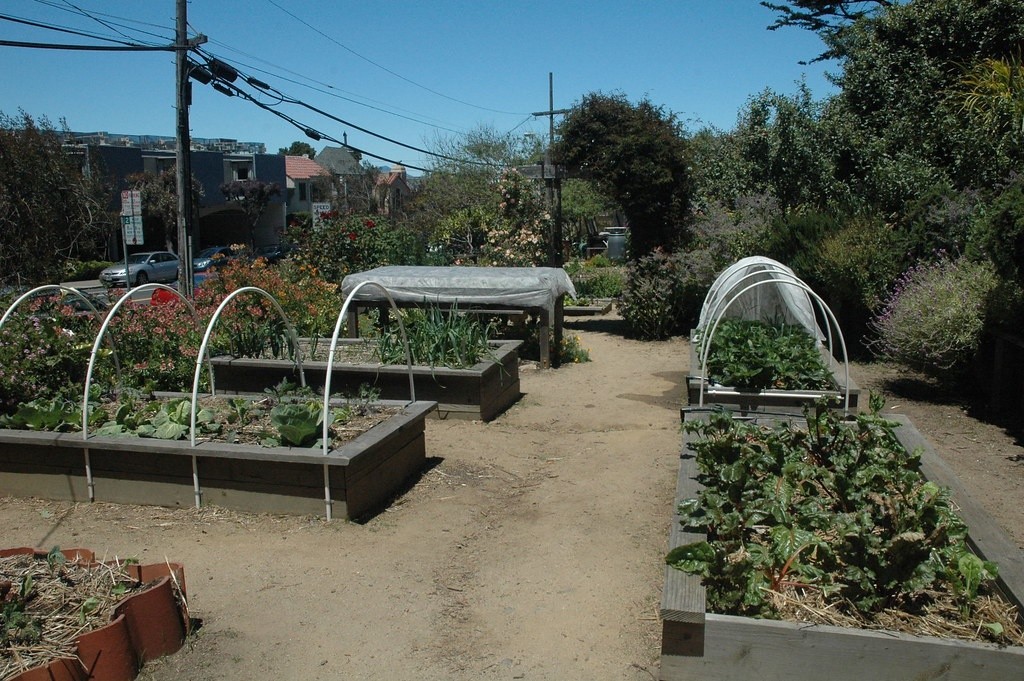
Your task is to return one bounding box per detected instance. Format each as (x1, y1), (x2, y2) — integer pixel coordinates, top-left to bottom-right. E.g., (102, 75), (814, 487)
(100, 250), (178, 286)
(193, 247), (239, 274)
(253, 245), (298, 265)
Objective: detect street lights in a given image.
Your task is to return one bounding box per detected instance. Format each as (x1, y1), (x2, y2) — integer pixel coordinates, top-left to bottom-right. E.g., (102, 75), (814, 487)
(525, 132), (563, 265)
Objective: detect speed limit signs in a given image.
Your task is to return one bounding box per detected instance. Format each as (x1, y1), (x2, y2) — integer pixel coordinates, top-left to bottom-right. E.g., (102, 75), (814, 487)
(313, 203), (332, 230)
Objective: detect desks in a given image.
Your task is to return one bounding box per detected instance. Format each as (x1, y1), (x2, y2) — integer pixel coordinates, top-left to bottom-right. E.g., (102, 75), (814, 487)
(341, 265), (578, 369)
